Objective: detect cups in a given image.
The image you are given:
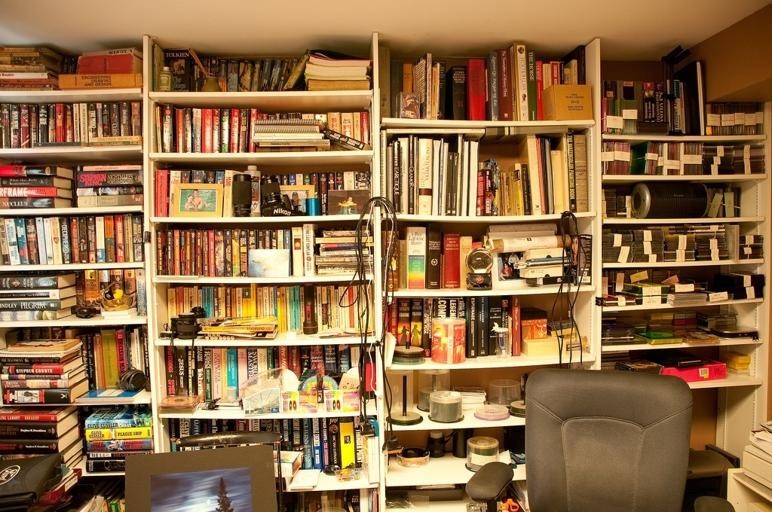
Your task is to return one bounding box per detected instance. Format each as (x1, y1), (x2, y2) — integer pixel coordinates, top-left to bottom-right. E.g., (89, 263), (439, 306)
(178, 313), (199, 339)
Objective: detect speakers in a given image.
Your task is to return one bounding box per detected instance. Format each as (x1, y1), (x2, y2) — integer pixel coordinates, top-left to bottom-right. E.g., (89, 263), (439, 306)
(629, 181), (708, 218)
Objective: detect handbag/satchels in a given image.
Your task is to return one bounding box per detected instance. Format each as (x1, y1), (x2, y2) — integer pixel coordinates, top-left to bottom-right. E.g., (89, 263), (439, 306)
(1, 454), (63, 511)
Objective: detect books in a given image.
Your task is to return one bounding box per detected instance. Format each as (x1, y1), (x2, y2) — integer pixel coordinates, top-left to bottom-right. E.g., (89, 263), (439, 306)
(1, 39), (155, 512)
(602, 62), (771, 490)
(152, 42), (379, 511)
(380, 43), (596, 363)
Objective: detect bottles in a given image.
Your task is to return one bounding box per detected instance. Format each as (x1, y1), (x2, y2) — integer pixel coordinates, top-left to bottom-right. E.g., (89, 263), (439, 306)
(233, 165), (262, 216)
(386, 371), (521, 473)
